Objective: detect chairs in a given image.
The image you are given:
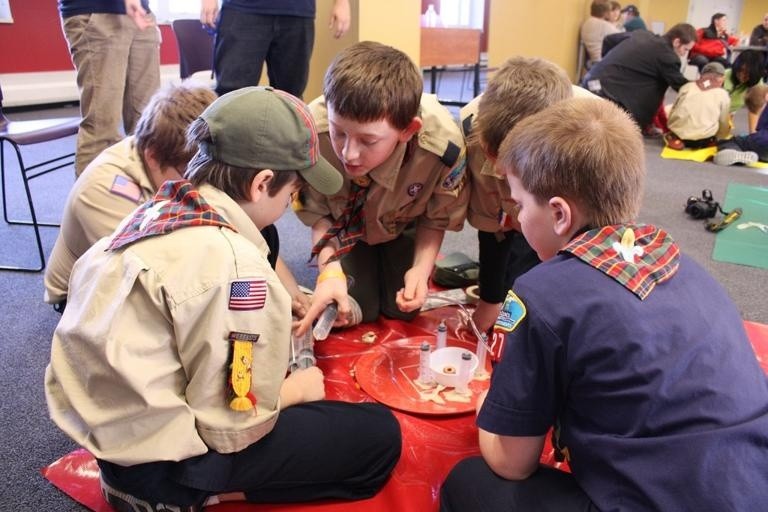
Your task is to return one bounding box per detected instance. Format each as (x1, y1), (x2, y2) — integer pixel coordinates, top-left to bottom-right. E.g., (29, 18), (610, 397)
(0, 85), (82, 272)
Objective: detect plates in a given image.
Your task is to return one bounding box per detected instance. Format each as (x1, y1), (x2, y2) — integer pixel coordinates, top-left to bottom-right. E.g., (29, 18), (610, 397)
(355, 334), (484, 418)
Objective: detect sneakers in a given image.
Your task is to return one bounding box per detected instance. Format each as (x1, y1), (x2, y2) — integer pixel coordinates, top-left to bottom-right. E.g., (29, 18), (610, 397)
(663, 131), (684, 149)
(714, 149), (758, 165)
(691, 136), (718, 149)
(95, 468), (209, 511)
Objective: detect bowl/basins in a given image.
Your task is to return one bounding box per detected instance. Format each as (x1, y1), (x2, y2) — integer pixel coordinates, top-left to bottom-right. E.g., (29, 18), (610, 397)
(427, 346), (479, 387)
(429, 346), (481, 391)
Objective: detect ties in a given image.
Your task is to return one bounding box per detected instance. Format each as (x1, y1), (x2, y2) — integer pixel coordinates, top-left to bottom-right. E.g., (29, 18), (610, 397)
(308, 178), (369, 261)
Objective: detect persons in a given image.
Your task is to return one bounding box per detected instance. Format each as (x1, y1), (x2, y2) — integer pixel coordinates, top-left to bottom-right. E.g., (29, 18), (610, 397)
(46, 86), (221, 315)
(44, 86), (403, 512)
(751, 15), (765, 50)
(440, 98), (766, 511)
(457, 59), (603, 333)
(713, 106), (768, 165)
(713, 51), (768, 135)
(289, 42), (473, 336)
(692, 13), (739, 70)
(670, 63), (733, 150)
(57, 0), (161, 180)
(199, 1), (350, 98)
(582, 0), (628, 68)
(581, 24), (697, 136)
(171, 3), (220, 84)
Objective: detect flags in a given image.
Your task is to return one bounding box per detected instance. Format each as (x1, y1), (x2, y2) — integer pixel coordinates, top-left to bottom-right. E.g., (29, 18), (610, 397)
(229, 281), (268, 309)
(110, 174), (141, 200)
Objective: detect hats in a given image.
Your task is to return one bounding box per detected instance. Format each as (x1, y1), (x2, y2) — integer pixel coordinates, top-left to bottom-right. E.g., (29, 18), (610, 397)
(198, 87), (345, 194)
(702, 61), (725, 75)
(621, 4), (640, 15)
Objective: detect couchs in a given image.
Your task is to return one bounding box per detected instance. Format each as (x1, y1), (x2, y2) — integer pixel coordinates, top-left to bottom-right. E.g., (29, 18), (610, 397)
(1, 62), (181, 108)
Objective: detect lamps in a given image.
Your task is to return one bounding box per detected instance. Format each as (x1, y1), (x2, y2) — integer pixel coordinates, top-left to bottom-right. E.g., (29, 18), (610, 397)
(1, 0), (15, 132)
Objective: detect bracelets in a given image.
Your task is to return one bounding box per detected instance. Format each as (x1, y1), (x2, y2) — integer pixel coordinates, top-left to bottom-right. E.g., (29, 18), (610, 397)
(315, 269), (348, 280)
